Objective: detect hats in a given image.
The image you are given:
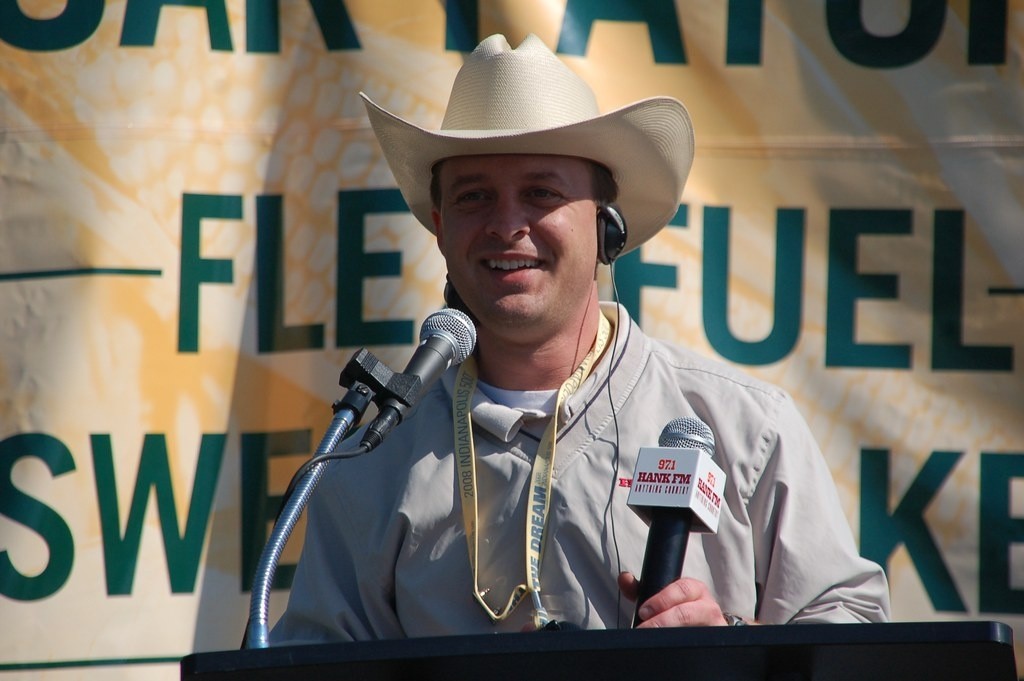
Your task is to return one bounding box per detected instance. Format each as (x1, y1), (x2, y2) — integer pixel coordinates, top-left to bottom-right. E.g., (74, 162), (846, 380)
(359, 33), (698, 260)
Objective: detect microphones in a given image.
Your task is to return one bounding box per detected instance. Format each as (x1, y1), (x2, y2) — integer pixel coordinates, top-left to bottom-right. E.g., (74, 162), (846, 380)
(356, 308), (478, 452)
(625, 416), (728, 629)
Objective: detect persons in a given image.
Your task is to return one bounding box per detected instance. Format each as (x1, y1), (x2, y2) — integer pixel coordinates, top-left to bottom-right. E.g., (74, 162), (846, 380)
(265, 32), (891, 652)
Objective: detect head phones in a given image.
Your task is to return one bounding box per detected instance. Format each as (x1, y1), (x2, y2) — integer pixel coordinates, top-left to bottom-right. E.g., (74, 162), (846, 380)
(441, 197), (627, 317)
